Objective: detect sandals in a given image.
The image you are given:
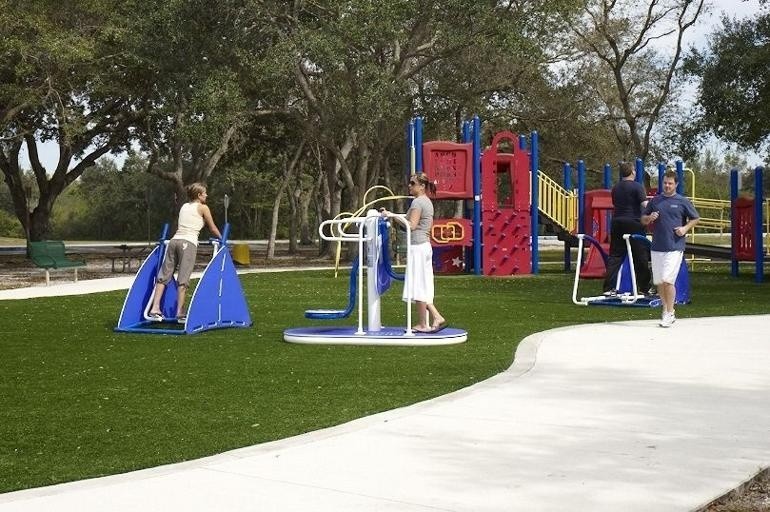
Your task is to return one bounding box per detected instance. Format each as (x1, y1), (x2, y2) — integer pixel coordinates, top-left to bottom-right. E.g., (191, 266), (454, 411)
(176, 314), (188, 323)
(430, 320), (449, 334)
(149, 311), (164, 318)
(404, 327), (430, 334)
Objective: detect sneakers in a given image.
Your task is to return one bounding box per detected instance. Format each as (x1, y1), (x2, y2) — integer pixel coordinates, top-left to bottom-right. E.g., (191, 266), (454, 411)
(604, 288), (619, 296)
(658, 308), (677, 328)
(639, 287), (656, 294)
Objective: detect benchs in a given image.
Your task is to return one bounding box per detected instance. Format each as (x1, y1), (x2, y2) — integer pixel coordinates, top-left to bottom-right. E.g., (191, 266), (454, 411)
(26, 240), (88, 285)
(97, 244), (254, 272)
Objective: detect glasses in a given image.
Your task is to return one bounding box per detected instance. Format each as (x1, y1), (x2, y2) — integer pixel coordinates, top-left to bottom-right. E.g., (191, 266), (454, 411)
(408, 180), (418, 185)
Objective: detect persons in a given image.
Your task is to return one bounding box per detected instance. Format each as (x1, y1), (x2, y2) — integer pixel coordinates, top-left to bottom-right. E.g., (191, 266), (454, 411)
(602, 160), (658, 297)
(148, 182), (225, 317)
(377, 171), (449, 334)
(640, 170), (700, 329)
